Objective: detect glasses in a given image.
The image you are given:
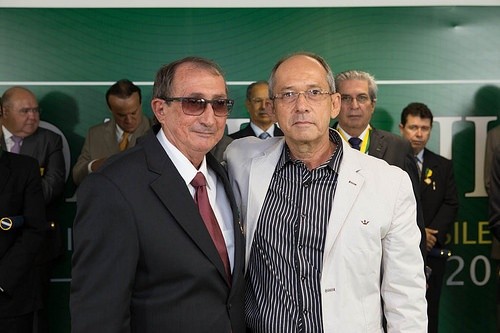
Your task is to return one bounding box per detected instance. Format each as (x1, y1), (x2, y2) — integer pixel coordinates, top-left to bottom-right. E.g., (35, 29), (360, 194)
(18, 107), (41, 115)
(341, 95), (367, 105)
(165, 97), (235, 117)
(270, 87), (335, 104)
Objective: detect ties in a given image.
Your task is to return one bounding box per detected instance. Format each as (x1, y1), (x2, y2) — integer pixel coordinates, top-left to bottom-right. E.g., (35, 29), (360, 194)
(190, 172), (231, 279)
(348, 137), (362, 151)
(118, 132), (129, 152)
(259, 132), (269, 140)
(414, 157), (422, 182)
(10, 136), (22, 154)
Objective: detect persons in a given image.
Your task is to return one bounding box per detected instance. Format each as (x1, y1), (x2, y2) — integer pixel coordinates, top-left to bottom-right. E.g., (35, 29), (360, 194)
(228, 80), (285, 140)
(399, 102), (460, 332)
(70, 56), (246, 332)
(220, 52), (428, 332)
(72, 79), (155, 185)
(330, 70), (421, 204)
(1, 150), (52, 333)
(484, 126), (499, 260)
(1, 87), (67, 218)
